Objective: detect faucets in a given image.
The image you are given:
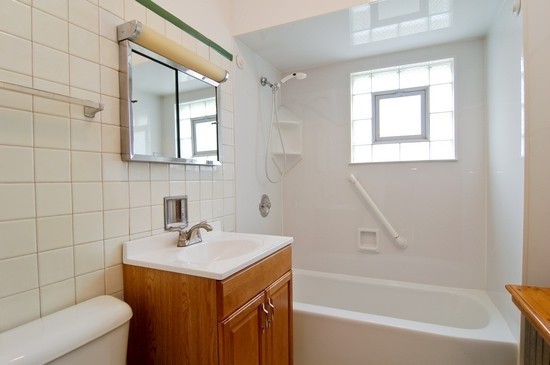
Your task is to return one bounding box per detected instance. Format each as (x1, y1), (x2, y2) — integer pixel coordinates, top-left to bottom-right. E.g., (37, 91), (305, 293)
(187, 223), (213, 241)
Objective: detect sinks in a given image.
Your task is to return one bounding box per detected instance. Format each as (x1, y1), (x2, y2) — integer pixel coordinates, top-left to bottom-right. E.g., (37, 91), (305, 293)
(121, 220), (295, 276)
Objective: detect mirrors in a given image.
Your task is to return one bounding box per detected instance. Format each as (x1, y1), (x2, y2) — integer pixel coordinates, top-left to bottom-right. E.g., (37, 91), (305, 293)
(120, 42), (222, 167)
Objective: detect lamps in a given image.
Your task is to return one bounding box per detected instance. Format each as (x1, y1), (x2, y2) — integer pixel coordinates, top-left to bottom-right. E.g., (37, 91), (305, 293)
(117, 19), (229, 83)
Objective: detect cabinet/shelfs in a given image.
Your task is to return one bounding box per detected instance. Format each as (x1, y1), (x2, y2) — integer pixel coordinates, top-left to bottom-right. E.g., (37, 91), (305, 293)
(273, 120), (302, 156)
(122, 244), (293, 365)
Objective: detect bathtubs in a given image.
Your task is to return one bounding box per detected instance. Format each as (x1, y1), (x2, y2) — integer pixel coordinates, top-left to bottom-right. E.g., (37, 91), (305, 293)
(292, 270), (518, 365)
